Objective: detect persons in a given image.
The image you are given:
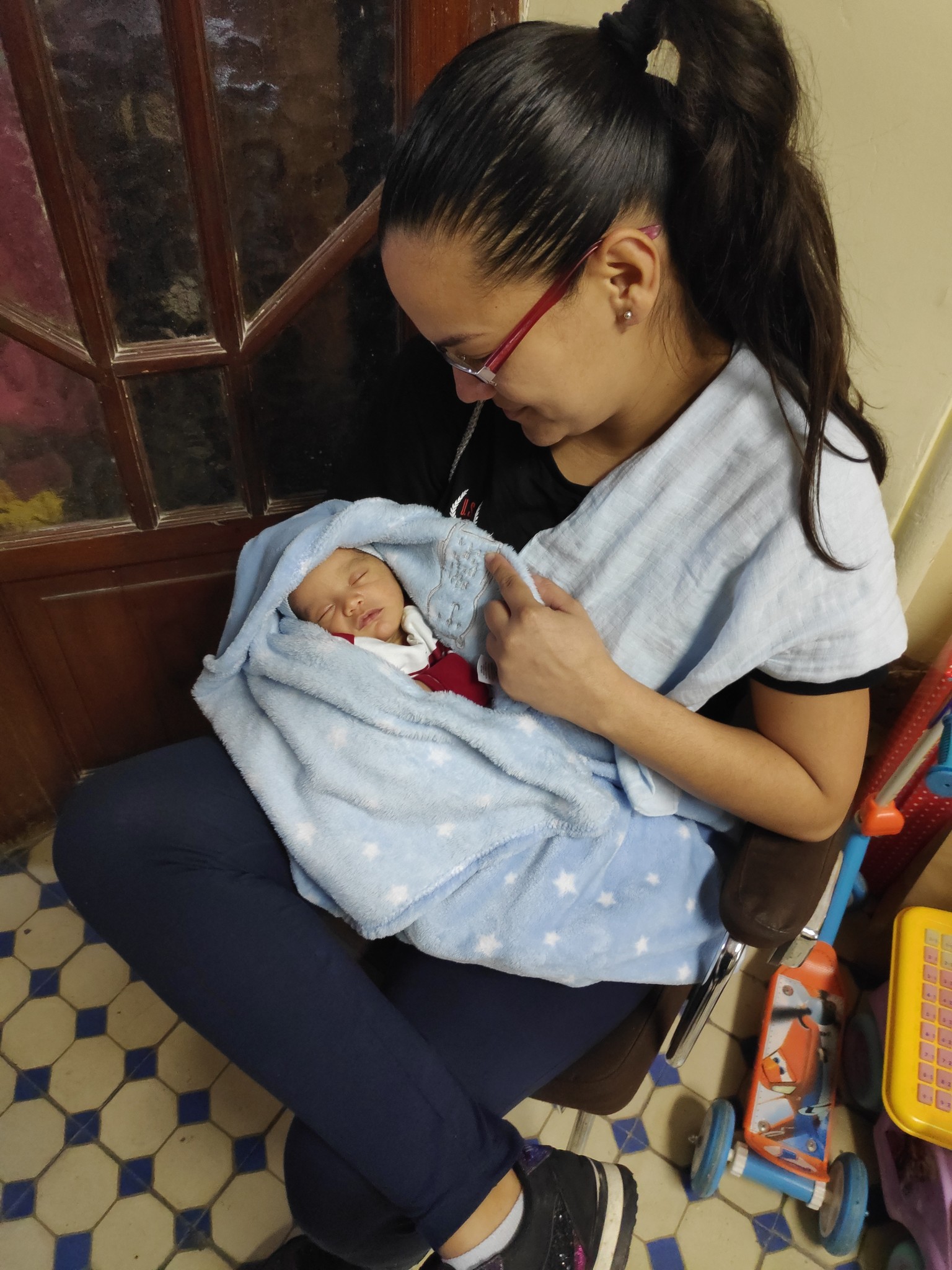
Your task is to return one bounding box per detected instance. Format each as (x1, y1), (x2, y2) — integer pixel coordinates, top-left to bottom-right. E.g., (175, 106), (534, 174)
(190, 499), (731, 988)
(51, 0), (911, 1270)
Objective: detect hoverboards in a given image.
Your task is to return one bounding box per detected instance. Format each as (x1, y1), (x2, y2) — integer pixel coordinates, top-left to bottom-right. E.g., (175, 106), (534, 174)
(688, 692), (952, 1259)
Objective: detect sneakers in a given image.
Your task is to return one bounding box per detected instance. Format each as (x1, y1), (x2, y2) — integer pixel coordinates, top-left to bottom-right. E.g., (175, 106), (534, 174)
(432, 1144), (639, 1270)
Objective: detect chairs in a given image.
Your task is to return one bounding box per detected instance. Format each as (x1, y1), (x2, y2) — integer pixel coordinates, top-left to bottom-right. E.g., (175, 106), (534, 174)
(531, 811), (845, 1154)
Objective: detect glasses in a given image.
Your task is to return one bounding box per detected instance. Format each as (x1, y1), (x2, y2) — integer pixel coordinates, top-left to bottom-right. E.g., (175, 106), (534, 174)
(433, 223), (661, 387)
